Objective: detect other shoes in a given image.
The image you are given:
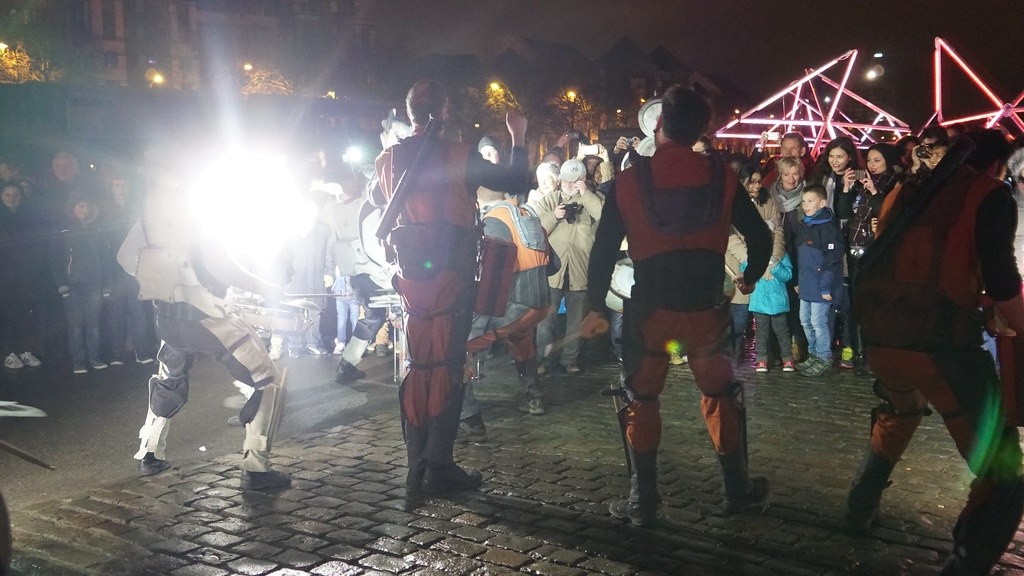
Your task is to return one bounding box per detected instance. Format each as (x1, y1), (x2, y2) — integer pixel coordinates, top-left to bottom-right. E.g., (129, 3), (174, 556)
(73, 359), (88, 373)
(110, 355), (123, 365)
(609, 499), (656, 525)
(333, 342), (345, 354)
(484, 349), (874, 376)
(845, 512), (876, 531)
(307, 345), (328, 354)
(722, 477), (768, 516)
(941, 558), (967, 576)
(422, 464), (471, 491)
(374, 345), (388, 357)
(88, 358), (108, 369)
(289, 347), (300, 357)
(395, 341), (399, 354)
(406, 469), (425, 488)
(136, 353), (154, 364)
(367, 340), (393, 350)
(268, 345), (282, 360)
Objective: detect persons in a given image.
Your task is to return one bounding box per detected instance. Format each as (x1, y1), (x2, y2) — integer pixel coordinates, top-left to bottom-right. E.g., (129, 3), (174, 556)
(116, 206), (295, 492)
(0, 124), (1024, 444)
(358, 79), (529, 506)
(851, 130), (1024, 576)
(577, 86), (774, 528)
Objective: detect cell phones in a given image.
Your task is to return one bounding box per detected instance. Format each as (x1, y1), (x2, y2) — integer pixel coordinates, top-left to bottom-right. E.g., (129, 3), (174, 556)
(625, 138), (635, 146)
(566, 132), (580, 140)
(764, 132), (780, 140)
(848, 170), (866, 180)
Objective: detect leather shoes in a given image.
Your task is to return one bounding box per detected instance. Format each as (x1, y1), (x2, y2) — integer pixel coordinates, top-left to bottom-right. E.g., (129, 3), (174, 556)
(241, 468), (292, 491)
(138, 453), (171, 477)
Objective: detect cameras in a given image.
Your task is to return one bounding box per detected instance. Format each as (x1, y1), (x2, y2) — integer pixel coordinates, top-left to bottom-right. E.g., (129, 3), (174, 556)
(916, 143), (934, 158)
(561, 202), (584, 224)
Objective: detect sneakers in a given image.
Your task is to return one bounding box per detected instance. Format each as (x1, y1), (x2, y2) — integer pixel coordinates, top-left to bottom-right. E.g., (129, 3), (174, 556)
(518, 397), (545, 414)
(453, 420), (488, 444)
(0, 352), (24, 369)
(18, 351), (41, 367)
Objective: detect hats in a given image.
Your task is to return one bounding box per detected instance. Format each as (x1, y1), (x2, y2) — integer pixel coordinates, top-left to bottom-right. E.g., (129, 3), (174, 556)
(559, 159), (587, 182)
(477, 136), (500, 153)
